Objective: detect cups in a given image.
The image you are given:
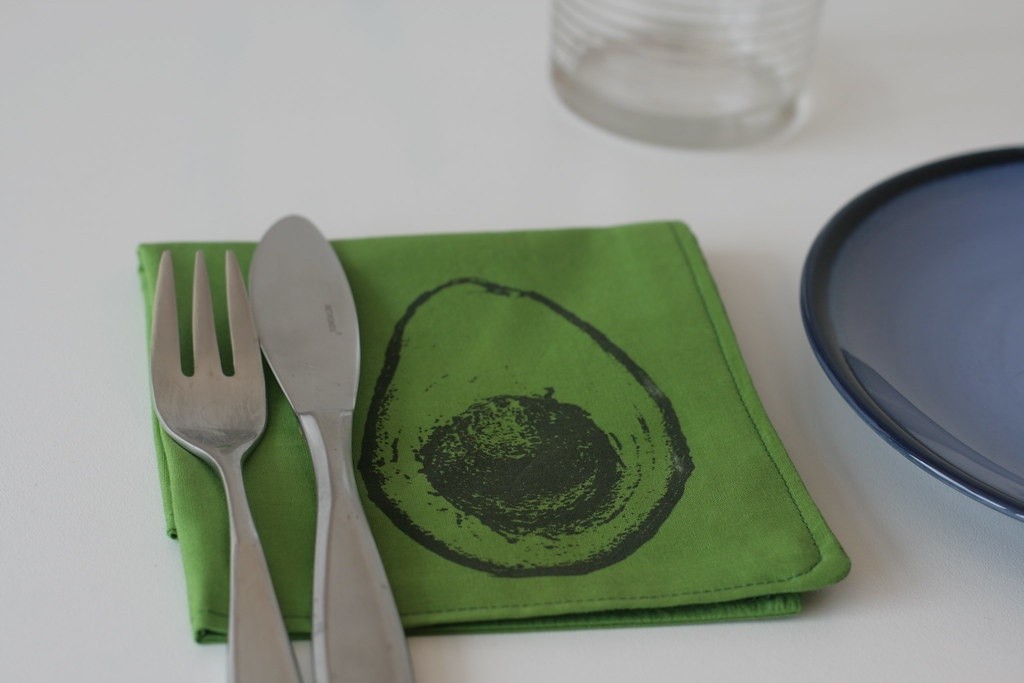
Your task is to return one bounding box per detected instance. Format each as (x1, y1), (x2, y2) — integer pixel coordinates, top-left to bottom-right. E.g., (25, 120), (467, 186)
(548, 1), (823, 148)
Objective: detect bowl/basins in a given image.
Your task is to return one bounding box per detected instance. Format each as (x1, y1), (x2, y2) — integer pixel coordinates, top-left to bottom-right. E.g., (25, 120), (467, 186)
(800, 145), (1024, 522)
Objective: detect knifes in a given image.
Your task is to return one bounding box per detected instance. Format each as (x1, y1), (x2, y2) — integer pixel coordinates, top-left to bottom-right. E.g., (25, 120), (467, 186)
(248, 215), (414, 683)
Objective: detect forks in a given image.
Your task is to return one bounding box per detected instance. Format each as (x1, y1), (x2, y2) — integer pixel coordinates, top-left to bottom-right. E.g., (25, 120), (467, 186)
(147, 246), (301, 683)
